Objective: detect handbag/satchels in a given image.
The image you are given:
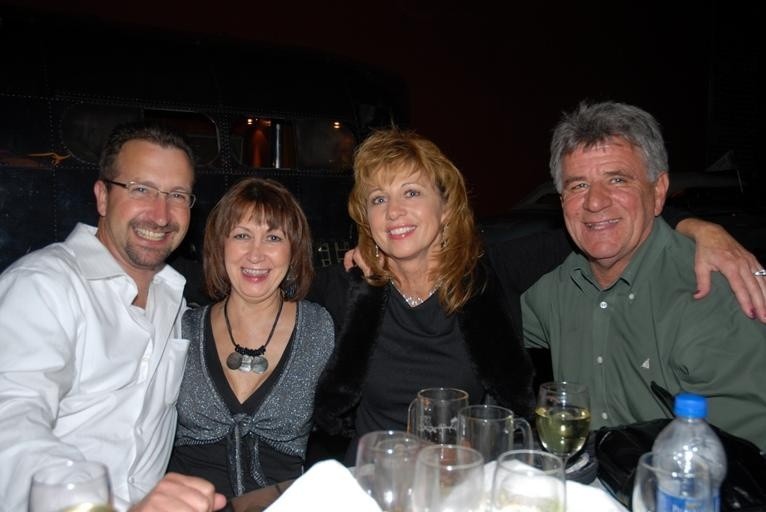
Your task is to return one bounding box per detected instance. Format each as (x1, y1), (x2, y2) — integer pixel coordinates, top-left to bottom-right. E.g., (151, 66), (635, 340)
(597, 418), (765, 512)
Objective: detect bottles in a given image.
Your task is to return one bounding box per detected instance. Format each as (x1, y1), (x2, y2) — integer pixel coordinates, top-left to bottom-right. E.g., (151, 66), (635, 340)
(649, 394), (726, 512)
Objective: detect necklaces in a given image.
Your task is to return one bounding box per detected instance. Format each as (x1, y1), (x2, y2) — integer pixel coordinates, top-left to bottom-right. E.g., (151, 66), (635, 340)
(220, 291), (290, 376)
(381, 268), (445, 308)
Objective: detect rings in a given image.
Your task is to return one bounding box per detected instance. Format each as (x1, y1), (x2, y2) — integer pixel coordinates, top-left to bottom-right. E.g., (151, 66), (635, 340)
(752, 269), (766, 276)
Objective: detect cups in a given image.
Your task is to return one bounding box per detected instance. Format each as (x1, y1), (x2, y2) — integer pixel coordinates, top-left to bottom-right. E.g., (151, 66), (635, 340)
(357, 429), (564, 512)
(26, 461), (111, 512)
(632, 449), (712, 511)
(409, 384), (535, 462)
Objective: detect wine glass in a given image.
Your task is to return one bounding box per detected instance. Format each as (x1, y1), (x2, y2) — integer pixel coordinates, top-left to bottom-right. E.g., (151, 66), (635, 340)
(536, 380), (591, 469)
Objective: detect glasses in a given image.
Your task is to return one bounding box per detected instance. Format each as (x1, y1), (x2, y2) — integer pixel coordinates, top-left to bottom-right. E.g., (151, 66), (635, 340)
(104, 179), (196, 208)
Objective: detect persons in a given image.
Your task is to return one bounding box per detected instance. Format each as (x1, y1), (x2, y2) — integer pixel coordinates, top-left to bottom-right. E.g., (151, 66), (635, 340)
(342, 103), (766, 455)
(173, 174), (337, 493)
(0, 126), (229, 512)
(312, 122), (766, 488)
